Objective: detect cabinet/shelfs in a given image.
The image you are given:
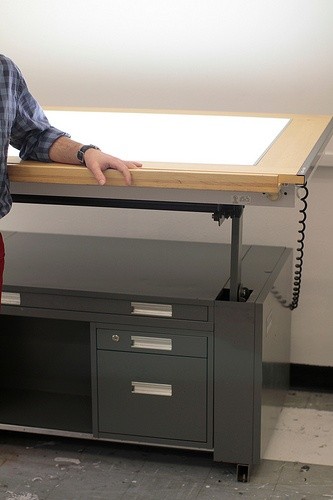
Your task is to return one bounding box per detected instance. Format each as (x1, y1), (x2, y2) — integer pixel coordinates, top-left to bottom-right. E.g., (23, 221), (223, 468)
(0, 107), (333, 483)
